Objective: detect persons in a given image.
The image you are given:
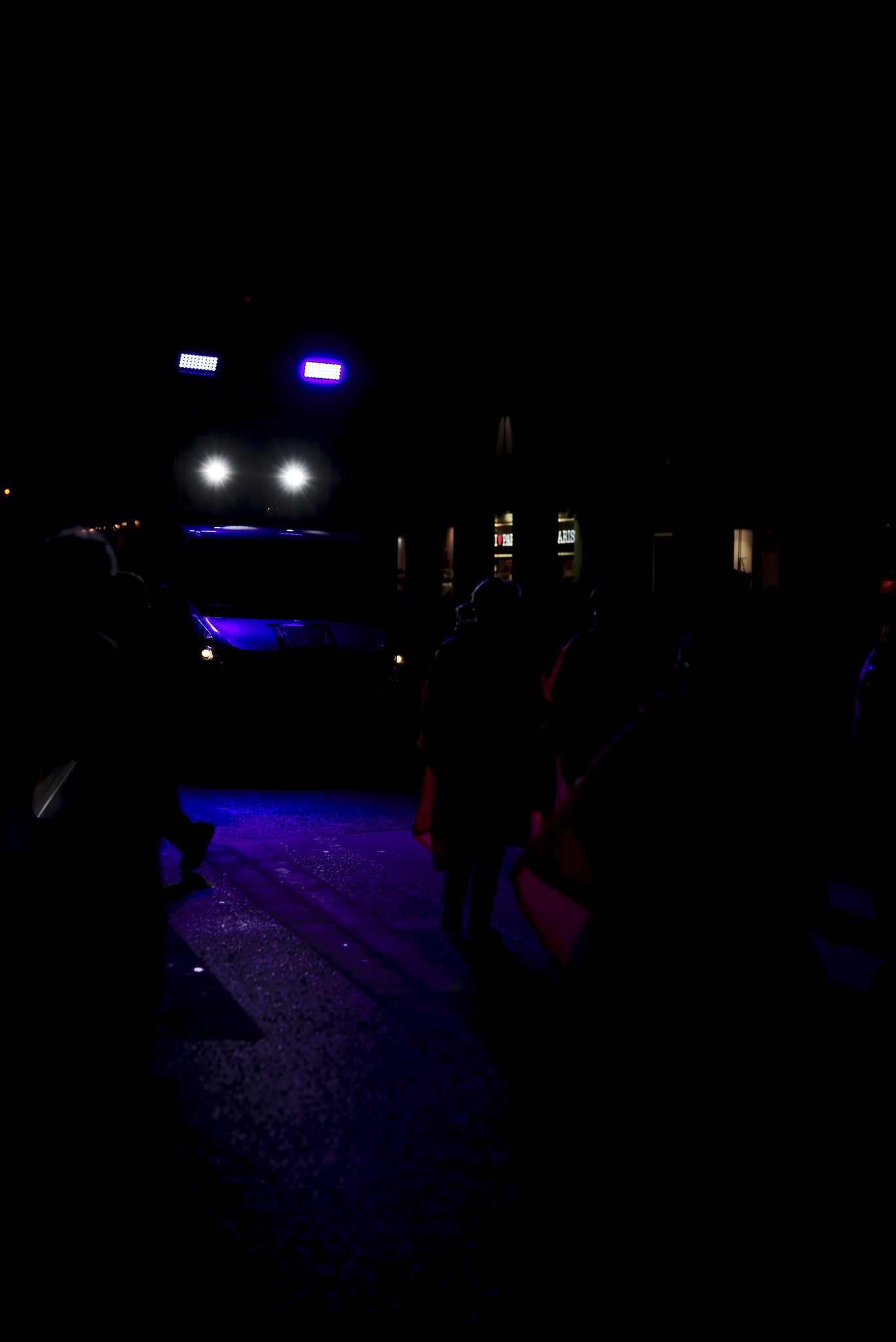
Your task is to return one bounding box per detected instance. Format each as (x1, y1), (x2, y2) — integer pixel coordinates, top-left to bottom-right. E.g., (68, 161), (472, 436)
(50, 529), (217, 894)
(416, 576), (895, 984)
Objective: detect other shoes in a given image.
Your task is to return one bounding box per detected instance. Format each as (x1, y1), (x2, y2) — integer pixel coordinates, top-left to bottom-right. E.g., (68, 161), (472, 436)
(441, 904), (462, 934)
(179, 823), (214, 873)
(469, 920), (502, 947)
